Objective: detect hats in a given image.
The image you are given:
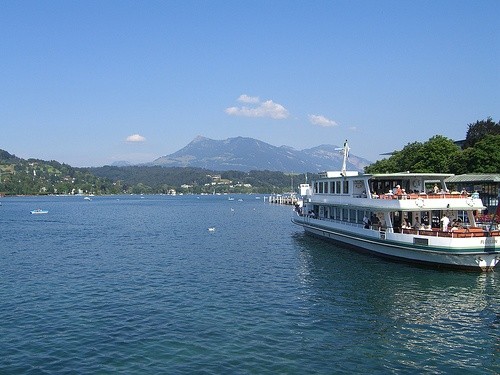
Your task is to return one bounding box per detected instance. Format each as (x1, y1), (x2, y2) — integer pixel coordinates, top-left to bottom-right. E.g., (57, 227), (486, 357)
(396, 185), (400, 188)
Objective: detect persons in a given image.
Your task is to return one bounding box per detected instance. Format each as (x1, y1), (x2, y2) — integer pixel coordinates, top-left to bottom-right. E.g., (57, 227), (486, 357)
(440, 214), (450, 232)
(394, 185), (406, 195)
(461, 188), (470, 195)
(414, 217), (421, 226)
(307, 210), (317, 219)
(433, 184), (439, 193)
(451, 219), (461, 230)
(363, 213), (380, 226)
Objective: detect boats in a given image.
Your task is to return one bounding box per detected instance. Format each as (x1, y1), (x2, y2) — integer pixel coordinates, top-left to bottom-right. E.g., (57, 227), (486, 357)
(290, 139), (500, 272)
(83, 197), (92, 201)
(29, 207), (48, 214)
